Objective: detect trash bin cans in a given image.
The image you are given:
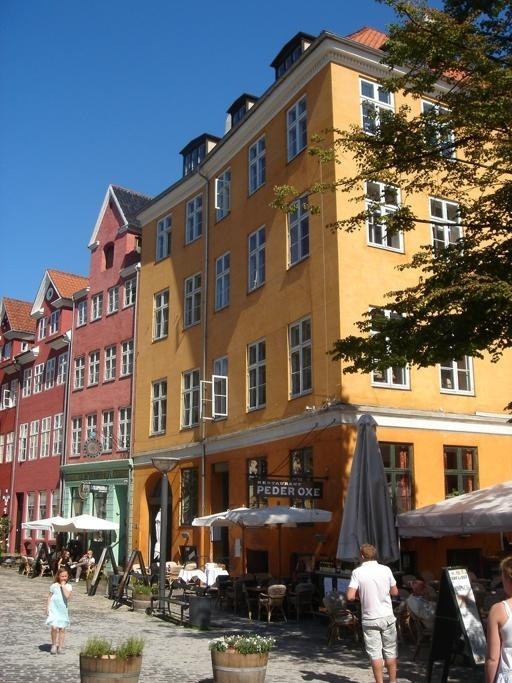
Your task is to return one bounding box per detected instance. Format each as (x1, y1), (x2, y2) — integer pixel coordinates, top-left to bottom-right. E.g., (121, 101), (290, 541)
(188, 596), (213, 625)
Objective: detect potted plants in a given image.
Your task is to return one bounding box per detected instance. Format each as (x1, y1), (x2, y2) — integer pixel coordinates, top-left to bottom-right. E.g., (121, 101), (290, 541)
(77, 635), (144, 683)
(131, 583), (157, 613)
(86, 569), (110, 595)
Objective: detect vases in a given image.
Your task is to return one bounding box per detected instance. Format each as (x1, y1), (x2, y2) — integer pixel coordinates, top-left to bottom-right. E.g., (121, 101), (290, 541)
(211, 647), (268, 682)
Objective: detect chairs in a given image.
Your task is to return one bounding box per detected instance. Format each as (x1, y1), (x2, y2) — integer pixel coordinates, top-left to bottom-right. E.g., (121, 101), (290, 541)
(21, 555), (35, 578)
(317, 592), (433, 662)
(210, 572), (315, 626)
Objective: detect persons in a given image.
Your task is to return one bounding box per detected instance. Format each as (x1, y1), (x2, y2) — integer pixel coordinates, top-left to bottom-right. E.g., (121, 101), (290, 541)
(346, 542), (400, 683)
(43, 567), (73, 654)
(485, 556), (512, 683)
(408, 579), (439, 630)
(70, 549), (96, 582)
(58, 550), (73, 582)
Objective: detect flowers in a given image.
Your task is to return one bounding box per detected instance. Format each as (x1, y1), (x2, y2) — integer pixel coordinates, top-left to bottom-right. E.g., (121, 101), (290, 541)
(205, 629), (277, 655)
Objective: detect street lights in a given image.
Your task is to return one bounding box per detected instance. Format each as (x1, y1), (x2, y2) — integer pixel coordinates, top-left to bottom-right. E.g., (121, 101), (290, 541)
(151, 456), (180, 613)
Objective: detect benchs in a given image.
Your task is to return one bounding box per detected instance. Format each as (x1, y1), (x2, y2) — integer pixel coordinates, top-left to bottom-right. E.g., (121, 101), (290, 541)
(146, 580), (210, 627)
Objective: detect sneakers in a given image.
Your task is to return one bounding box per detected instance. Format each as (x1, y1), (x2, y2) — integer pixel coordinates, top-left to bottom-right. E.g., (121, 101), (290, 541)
(50, 645), (66, 654)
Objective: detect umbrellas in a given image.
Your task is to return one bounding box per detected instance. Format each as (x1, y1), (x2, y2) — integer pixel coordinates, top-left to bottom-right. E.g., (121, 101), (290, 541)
(334, 413), (400, 565)
(192, 504), (293, 580)
(50, 512), (119, 552)
(21, 510), (68, 554)
(221, 506), (333, 583)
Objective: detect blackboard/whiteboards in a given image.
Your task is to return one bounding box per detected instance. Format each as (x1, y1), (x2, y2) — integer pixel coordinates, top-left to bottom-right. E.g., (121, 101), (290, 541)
(31, 542), (44, 569)
(445, 566), (487, 666)
(90, 546), (108, 586)
(115, 549), (138, 598)
